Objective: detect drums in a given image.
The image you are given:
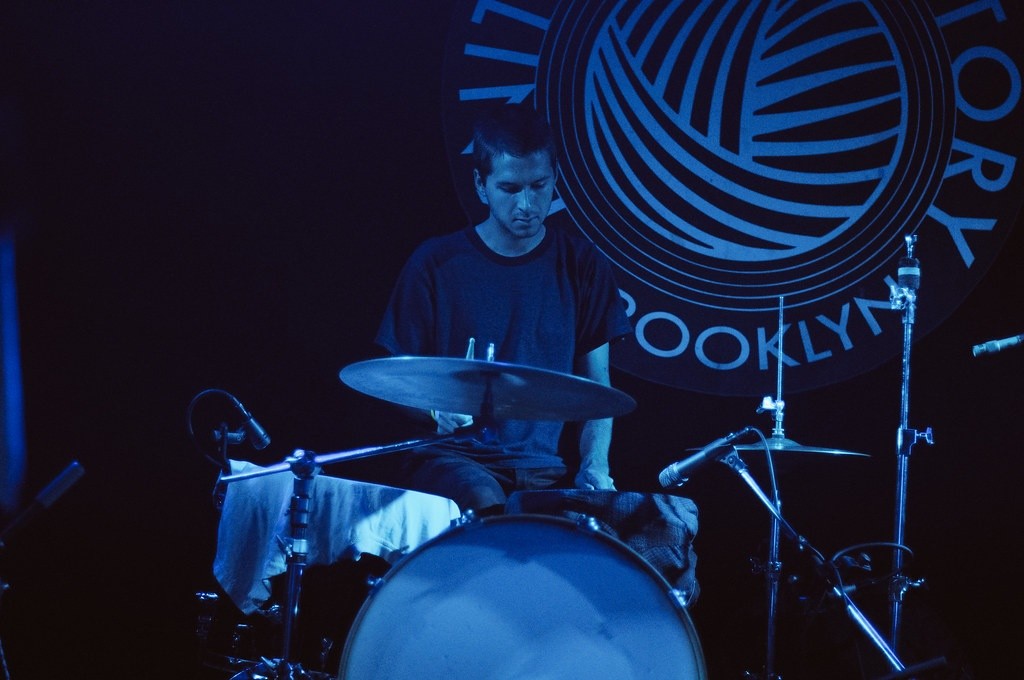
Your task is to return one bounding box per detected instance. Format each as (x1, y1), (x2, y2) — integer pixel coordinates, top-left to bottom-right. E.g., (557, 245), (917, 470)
(506, 485), (701, 605)
(337, 492), (710, 680)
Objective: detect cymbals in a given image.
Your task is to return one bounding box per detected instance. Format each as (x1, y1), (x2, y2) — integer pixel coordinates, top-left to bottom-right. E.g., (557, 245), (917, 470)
(685, 433), (871, 458)
(337, 355), (638, 425)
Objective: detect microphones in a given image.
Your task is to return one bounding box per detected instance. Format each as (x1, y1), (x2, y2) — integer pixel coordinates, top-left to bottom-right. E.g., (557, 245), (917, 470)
(659, 427), (751, 490)
(225, 394), (272, 451)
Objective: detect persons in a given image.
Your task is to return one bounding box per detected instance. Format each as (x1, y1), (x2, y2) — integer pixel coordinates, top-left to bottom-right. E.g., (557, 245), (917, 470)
(383, 103), (622, 527)
